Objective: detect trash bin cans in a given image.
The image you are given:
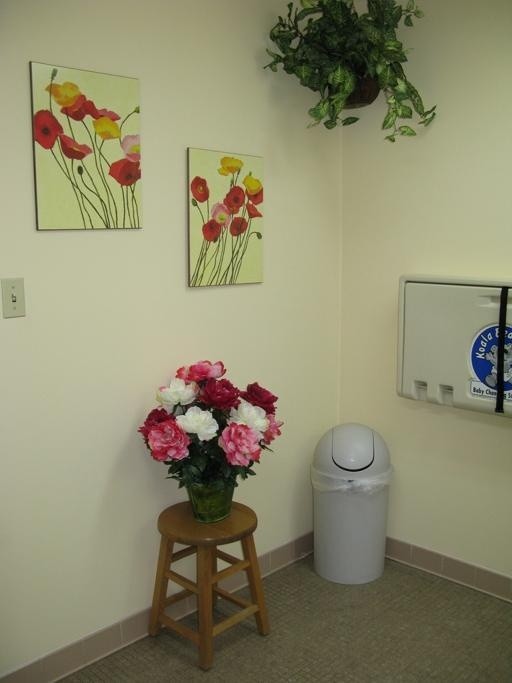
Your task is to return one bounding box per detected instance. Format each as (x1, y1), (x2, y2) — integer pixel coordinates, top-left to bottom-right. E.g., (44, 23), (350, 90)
(310, 422), (392, 585)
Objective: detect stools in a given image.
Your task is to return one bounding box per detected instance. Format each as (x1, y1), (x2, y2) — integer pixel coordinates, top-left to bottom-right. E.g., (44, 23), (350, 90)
(150, 504), (270, 671)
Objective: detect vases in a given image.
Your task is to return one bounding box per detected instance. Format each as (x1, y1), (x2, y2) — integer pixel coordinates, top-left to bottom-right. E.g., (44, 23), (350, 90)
(187, 468), (233, 522)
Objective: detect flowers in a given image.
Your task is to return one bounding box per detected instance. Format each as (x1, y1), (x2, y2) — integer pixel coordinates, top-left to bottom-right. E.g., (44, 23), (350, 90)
(137, 359), (283, 489)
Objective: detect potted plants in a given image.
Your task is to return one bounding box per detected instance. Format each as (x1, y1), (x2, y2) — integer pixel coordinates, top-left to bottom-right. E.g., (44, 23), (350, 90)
(262, 0), (436, 141)
